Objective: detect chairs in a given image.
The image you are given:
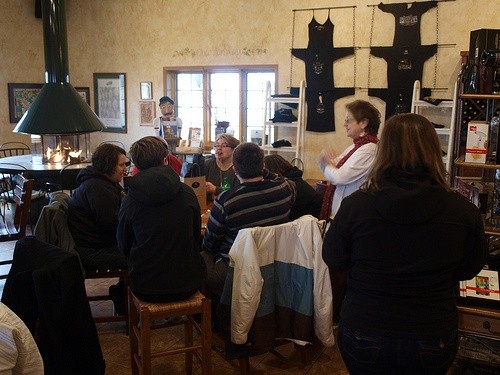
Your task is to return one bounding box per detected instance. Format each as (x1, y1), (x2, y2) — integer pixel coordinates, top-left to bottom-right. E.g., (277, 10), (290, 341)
(32, 191), (127, 322)
(0, 142), (31, 157)
(0, 173), (34, 278)
(211, 214), (335, 375)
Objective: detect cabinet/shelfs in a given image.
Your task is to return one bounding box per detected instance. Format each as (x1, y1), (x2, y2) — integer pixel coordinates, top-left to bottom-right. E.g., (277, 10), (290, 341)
(259, 80), (307, 169)
(453, 52), (500, 235)
(411, 80), (458, 186)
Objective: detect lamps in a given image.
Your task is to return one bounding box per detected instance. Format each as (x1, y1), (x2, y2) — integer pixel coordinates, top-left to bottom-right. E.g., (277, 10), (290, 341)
(12, 0), (105, 136)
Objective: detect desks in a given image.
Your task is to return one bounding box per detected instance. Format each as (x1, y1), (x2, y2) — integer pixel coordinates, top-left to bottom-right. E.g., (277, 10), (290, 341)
(456, 304), (500, 340)
(0, 153), (131, 191)
(176, 147), (203, 163)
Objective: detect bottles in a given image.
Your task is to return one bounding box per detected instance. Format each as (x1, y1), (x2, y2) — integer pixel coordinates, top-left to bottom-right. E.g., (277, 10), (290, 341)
(221, 177), (230, 192)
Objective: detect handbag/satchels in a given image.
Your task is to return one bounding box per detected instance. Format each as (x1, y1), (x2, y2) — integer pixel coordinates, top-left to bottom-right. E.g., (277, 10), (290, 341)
(291, 158), (304, 177)
(180, 163), (207, 210)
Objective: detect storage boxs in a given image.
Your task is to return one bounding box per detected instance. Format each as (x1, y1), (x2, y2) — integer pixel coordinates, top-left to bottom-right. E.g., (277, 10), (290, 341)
(465, 121), (489, 164)
(454, 176), (494, 232)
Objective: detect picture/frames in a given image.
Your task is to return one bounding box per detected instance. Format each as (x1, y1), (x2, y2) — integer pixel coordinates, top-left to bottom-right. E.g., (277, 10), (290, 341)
(140, 81), (153, 99)
(138, 101), (156, 126)
(7, 82), (90, 124)
(93, 72), (129, 134)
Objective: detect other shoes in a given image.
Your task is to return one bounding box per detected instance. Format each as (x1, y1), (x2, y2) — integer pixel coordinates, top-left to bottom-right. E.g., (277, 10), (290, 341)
(194, 315), (221, 333)
(109, 285), (125, 315)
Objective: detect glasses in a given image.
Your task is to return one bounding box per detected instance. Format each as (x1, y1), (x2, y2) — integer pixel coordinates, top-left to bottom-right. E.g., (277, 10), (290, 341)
(215, 142), (231, 149)
(344, 117), (355, 124)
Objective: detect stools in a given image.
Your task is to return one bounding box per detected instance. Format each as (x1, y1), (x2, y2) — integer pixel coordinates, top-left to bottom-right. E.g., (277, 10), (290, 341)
(127, 287), (212, 375)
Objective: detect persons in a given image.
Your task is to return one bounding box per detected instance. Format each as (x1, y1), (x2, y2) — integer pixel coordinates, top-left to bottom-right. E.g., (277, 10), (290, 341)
(322, 113), (489, 375)
(67, 134), (320, 316)
(318, 100), (381, 239)
(153, 95), (182, 141)
